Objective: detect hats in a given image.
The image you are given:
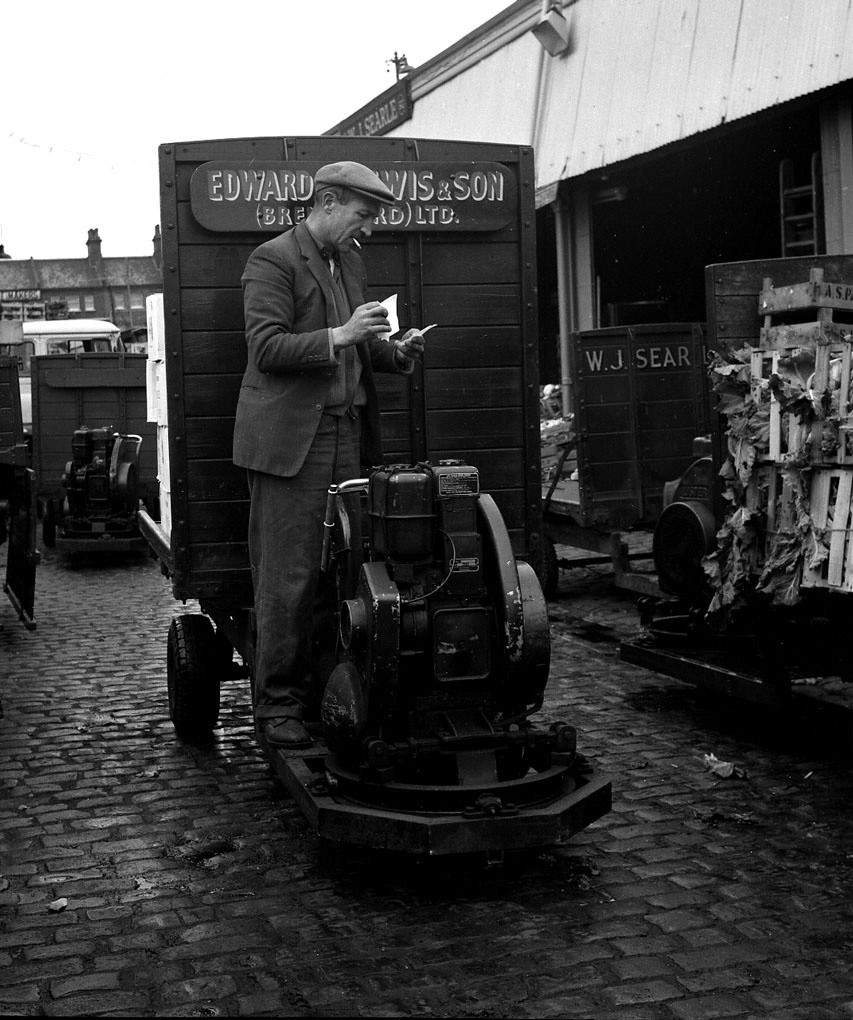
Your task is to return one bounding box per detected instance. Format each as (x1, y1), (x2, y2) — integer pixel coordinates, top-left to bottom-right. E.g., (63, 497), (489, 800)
(315, 160), (396, 207)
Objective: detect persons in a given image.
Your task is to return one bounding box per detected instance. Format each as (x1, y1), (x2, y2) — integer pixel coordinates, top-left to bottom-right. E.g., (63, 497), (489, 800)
(233, 159), (426, 748)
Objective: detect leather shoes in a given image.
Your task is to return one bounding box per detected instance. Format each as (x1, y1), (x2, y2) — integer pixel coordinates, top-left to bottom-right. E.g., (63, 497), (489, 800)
(257, 715), (310, 748)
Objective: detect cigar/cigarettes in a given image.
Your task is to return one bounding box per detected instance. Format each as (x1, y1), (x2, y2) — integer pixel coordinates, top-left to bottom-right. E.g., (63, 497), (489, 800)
(352, 238), (361, 249)
